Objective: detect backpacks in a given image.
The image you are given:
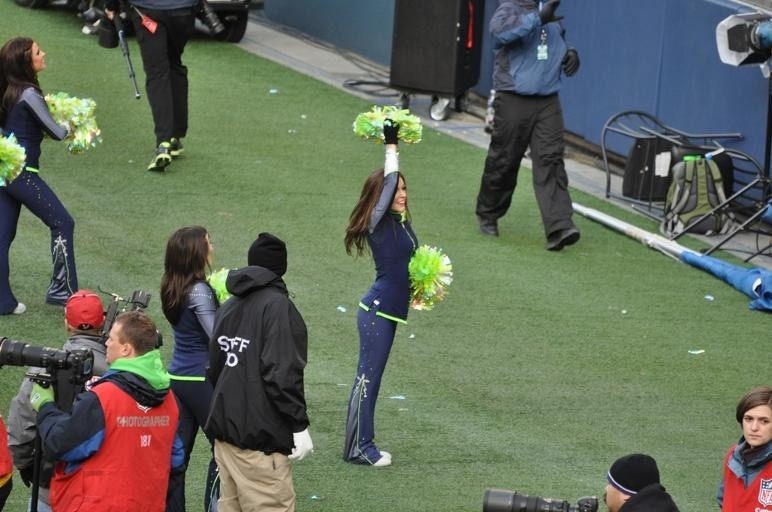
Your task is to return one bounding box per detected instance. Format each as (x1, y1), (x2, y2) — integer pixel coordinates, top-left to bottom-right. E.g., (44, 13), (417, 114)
(659, 159), (744, 239)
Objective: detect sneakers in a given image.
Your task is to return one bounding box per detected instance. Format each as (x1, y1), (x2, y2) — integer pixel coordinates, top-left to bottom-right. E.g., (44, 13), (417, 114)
(13, 303), (27, 315)
(147, 141), (172, 172)
(373, 451), (393, 466)
(479, 215), (499, 236)
(169, 137), (185, 156)
(547, 226), (579, 251)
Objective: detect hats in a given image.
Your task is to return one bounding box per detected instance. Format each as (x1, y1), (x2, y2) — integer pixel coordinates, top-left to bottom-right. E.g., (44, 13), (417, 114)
(606, 454), (659, 496)
(248, 232), (287, 275)
(66, 290), (106, 332)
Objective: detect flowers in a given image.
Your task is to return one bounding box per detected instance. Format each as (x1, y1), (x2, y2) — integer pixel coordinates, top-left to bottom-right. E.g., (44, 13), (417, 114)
(205, 267), (233, 302)
(0, 132), (42, 191)
(352, 106), (423, 144)
(43, 94), (103, 153)
(408, 244), (453, 311)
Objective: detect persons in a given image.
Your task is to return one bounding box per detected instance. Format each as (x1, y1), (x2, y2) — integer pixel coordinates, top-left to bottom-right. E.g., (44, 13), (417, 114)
(475, 0), (581, 251)
(160, 227), (309, 512)
(715, 386), (772, 512)
(603, 454), (681, 512)
(343, 117), (418, 468)
(104, 0), (201, 174)
(0, 37), (78, 315)
(1, 287), (186, 512)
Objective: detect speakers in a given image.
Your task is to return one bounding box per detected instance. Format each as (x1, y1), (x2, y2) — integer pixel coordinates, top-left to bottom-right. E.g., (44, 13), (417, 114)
(388, 1), (484, 112)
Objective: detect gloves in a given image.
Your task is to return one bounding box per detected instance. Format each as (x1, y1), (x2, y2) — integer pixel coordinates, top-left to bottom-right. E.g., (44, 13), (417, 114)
(287, 428), (314, 461)
(562, 48), (580, 77)
(538, 1), (564, 25)
(29, 381), (56, 414)
(385, 118), (398, 144)
